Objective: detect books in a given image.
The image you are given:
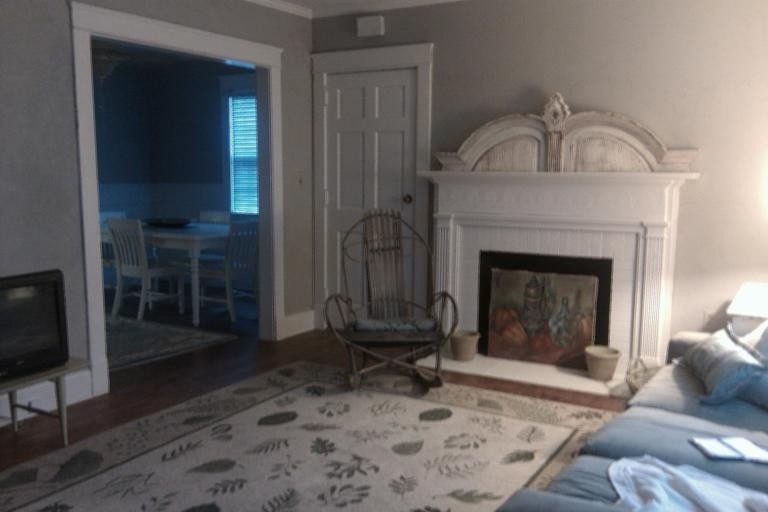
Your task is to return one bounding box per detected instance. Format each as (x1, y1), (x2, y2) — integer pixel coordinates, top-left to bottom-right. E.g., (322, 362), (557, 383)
(689, 436), (768, 464)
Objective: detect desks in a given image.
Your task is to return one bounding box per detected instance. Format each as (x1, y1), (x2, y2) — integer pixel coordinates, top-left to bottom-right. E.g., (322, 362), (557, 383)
(1, 359), (91, 448)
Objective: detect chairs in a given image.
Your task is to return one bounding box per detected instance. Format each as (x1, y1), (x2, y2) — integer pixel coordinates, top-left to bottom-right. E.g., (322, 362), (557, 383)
(321, 205), (459, 389)
(104, 218), (186, 320)
(198, 212), (257, 323)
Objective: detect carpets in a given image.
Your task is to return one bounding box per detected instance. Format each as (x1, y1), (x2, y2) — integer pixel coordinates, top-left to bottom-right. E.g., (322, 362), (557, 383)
(0, 358), (618, 510)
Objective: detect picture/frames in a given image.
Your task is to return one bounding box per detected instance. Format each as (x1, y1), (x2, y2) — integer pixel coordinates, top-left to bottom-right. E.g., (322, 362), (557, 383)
(475, 249), (614, 373)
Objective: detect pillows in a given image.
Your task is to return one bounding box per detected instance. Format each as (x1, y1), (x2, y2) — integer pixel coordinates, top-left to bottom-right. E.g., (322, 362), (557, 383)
(671, 320), (768, 406)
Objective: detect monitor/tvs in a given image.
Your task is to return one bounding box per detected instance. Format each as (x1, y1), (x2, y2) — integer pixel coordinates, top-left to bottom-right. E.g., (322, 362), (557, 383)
(1, 269), (70, 385)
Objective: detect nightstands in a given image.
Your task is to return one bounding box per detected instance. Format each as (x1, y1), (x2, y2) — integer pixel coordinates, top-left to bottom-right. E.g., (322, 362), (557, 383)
(726, 279), (768, 321)
